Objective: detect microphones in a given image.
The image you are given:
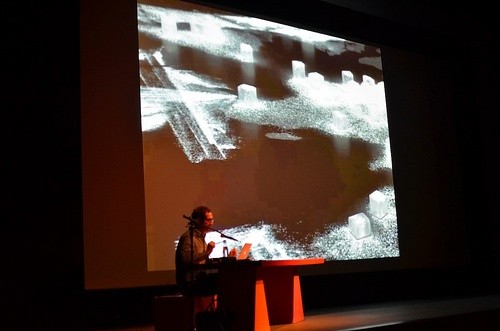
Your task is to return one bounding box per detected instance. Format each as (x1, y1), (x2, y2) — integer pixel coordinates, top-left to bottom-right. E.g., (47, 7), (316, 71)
(221, 234), (225, 237)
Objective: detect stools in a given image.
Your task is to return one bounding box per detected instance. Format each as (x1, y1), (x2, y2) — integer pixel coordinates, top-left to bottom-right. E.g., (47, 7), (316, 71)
(153, 295), (192, 331)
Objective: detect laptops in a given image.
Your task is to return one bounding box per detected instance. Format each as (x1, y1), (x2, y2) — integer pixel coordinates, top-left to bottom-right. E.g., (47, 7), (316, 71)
(237, 243), (252, 259)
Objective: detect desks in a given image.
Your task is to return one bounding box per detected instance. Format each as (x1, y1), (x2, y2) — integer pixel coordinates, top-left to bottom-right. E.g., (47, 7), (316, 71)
(254, 259), (324, 331)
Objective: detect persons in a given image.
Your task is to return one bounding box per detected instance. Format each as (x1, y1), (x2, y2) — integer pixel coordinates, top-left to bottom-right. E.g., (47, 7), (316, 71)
(176, 206), (216, 331)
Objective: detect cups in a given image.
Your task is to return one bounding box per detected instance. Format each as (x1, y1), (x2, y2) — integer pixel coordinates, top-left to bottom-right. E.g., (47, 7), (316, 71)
(223, 247), (228, 257)
